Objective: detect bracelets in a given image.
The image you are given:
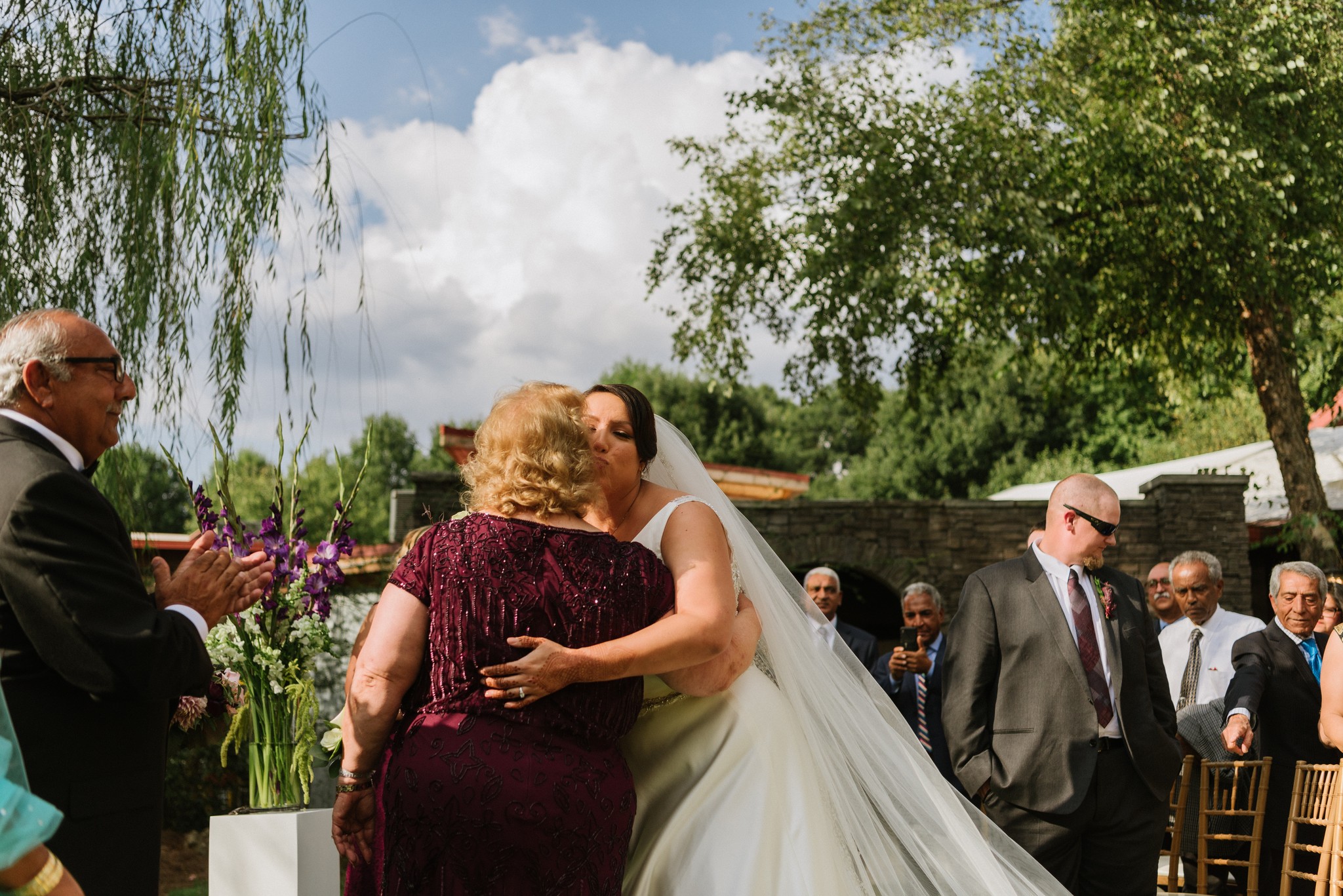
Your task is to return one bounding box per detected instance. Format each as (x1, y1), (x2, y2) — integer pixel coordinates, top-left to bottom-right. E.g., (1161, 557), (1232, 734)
(339, 767), (376, 779)
(336, 781), (373, 793)
(1, 848), (64, 896)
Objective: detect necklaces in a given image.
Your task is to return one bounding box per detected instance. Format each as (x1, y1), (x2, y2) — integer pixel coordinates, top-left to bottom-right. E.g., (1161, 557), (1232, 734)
(611, 480), (641, 535)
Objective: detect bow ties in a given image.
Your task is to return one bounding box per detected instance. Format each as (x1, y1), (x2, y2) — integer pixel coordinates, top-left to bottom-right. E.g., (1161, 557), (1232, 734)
(81, 459), (100, 479)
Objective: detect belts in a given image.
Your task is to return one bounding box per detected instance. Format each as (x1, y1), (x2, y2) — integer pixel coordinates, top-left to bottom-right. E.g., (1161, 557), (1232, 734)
(1097, 736), (1125, 752)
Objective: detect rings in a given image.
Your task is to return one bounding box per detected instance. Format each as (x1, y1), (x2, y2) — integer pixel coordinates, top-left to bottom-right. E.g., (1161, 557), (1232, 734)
(518, 686), (525, 698)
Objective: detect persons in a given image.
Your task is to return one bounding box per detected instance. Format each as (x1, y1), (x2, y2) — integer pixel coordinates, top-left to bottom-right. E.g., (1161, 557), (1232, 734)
(941, 474), (1181, 896)
(1147, 561), (1188, 637)
(1313, 582), (1343, 636)
(1027, 522), (1046, 550)
(1318, 621), (1343, 757)
(330, 380), (762, 896)
(344, 383), (1076, 896)
(802, 566), (881, 674)
(1, 309), (275, 896)
(870, 582), (981, 812)
(1158, 550), (1267, 893)
(1, 683), (90, 896)
(1221, 560), (1343, 896)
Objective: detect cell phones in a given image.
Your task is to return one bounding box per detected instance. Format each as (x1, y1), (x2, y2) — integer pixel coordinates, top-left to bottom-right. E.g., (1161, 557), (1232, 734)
(900, 627), (919, 652)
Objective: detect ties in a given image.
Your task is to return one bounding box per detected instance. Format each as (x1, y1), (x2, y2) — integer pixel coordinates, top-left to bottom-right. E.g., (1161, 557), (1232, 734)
(1067, 568), (1113, 729)
(1176, 628), (1203, 712)
(817, 627), (828, 644)
(918, 674), (931, 750)
(1301, 638), (1320, 684)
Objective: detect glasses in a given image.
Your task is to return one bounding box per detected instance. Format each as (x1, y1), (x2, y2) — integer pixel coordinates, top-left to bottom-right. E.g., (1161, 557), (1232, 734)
(54, 355), (126, 385)
(1063, 503), (1119, 536)
(1322, 606), (1341, 619)
(1142, 578), (1171, 593)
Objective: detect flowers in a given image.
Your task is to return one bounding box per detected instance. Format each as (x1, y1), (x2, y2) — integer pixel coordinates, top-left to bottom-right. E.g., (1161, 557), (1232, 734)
(151, 409), (376, 809)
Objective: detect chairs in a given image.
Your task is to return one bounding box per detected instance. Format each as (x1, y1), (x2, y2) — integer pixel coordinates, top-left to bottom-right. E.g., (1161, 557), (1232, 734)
(1153, 752), (1343, 896)
